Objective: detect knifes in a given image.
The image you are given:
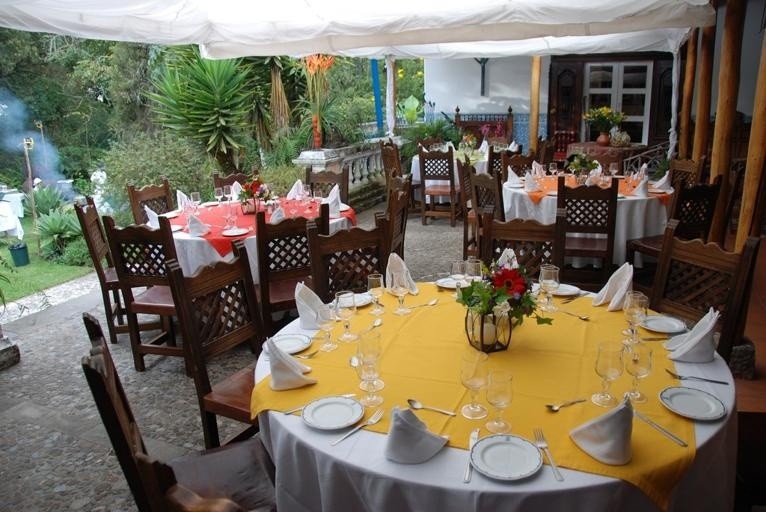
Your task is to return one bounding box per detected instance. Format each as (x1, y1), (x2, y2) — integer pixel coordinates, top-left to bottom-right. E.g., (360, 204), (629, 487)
(640, 337), (670, 343)
(631, 409), (687, 447)
(285, 392), (357, 415)
(561, 292), (591, 305)
(461, 425), (482, 484)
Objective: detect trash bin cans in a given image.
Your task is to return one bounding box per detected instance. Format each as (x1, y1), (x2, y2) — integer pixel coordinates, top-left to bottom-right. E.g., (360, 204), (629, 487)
(8, 242), (29, 267)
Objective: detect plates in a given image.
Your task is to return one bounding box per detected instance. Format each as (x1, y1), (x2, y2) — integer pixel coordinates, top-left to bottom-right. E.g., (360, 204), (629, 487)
(660, 386), (728, 421)
(156, 184), (350, 236)
(640, 314), (688, 333)
(548, 283), (581, 296)
(436, 276), (471, 290)
(504, 163), (666, 195)
(470, 433), (548, 482)
(355, 293), (372, 308)
(262, 332), (312, 354)
(301, 396), (373, 431)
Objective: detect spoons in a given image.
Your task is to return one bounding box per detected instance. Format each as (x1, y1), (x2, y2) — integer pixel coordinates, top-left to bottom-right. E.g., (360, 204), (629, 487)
(559, 309), (591, 320)
(358, 318), (385, 338)
(408, 398), (457, 416)
(545, 397), (588, 412)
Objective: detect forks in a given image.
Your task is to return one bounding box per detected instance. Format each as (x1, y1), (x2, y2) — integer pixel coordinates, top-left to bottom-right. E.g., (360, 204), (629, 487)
(266, 350), (319, 363)
(328, 409), (385, 446)
(664, 367), (730, 385)
(408, 297), (440, 310)
(533, 428), (563, 481)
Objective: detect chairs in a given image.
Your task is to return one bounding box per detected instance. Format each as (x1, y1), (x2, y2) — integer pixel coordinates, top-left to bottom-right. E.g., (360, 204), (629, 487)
(456, 153), (496, 264)
(379, 127), (745, 228)
(75, 164), (413, 373)
(161, 236), (274, 452)
(624, 174), (724, 304)
(74, 312), (279, 509)
(469, 164), (503, 260)
(134, 446), (241, 510)
(480, 210), (569, 283)
(649, 219), (762, 367)
(554, 174), (620, 297)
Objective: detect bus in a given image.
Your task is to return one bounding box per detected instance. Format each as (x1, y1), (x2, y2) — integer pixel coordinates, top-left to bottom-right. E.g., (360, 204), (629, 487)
(561, 70), (646, 136)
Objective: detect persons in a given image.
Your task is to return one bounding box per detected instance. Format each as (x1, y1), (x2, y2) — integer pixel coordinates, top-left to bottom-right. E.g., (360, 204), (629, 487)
(22, 171), (35, 193)
(90, 161), (107, 195)
(33, 178), (43, 192)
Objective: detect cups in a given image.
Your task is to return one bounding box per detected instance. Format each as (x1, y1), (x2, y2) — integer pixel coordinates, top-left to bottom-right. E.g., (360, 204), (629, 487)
(467, 260), (484, 283)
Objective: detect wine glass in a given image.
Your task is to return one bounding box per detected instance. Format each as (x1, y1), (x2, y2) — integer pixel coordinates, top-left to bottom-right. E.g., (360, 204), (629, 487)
(316, 289), (358, 354)
(590, 342), (624, 406)
(450, 259), (467, 298)
(429, 142), (510, 157)
(625, 342), (654, 404)
(622, 289), (649, 345)
(353, 330), (386, 406)
(460, 349), (516, 433)
(367, 270), (413, 316)
(539, 264), (559, 313)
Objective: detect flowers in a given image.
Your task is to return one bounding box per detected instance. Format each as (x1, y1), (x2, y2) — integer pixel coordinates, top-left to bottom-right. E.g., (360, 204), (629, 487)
(452, 246), (554, 329)
(581, 106), (629, 131)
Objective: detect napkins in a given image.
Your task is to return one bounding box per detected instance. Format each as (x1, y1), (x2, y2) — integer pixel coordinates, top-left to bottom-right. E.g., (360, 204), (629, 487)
(568, 393), (635, 465)
(664, 306), (721, 364)
(592, 262), (634, 312)
(265, 336), (318, 393)
(384, 407), (450, 465)
